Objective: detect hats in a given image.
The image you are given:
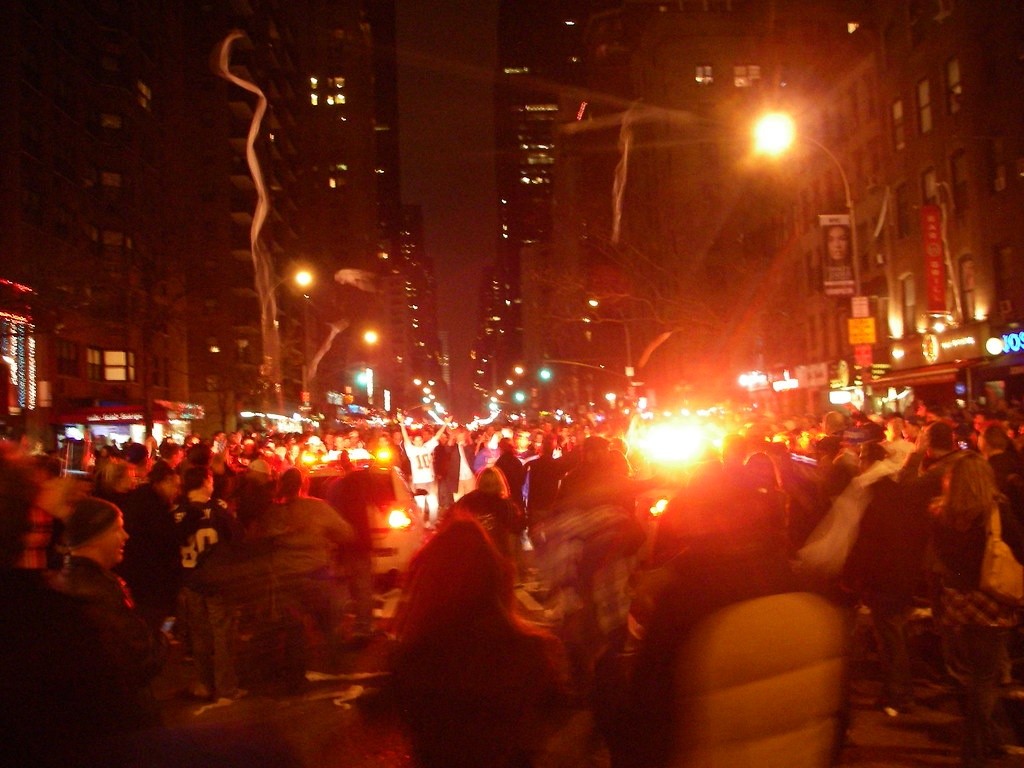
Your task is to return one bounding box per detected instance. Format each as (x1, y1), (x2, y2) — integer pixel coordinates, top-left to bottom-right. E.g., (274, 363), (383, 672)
(60, 493), (123, 549)
(249, 459), (271, 476)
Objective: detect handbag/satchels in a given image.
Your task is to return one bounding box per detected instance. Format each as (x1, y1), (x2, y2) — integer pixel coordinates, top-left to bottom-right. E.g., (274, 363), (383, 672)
(978, 497), (1024, 607)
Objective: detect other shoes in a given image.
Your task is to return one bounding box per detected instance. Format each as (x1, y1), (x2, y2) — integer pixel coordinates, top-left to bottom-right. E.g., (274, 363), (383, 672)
(230, 689), (248, 700)
(188, 683), (210, 698)
(862, 687), (912, 714)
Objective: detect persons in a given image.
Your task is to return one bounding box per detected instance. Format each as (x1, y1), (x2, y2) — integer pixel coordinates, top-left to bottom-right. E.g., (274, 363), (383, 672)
(1, 401), (1024, 768)
(389, 519), (572, 768)
(929, 456), (1023, 768)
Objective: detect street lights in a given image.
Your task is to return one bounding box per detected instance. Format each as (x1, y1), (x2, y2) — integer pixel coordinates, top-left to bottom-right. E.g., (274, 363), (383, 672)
(261, 269), (311, 415)
(751, 111), (868, 383)
(588, 298), (637, 402)
